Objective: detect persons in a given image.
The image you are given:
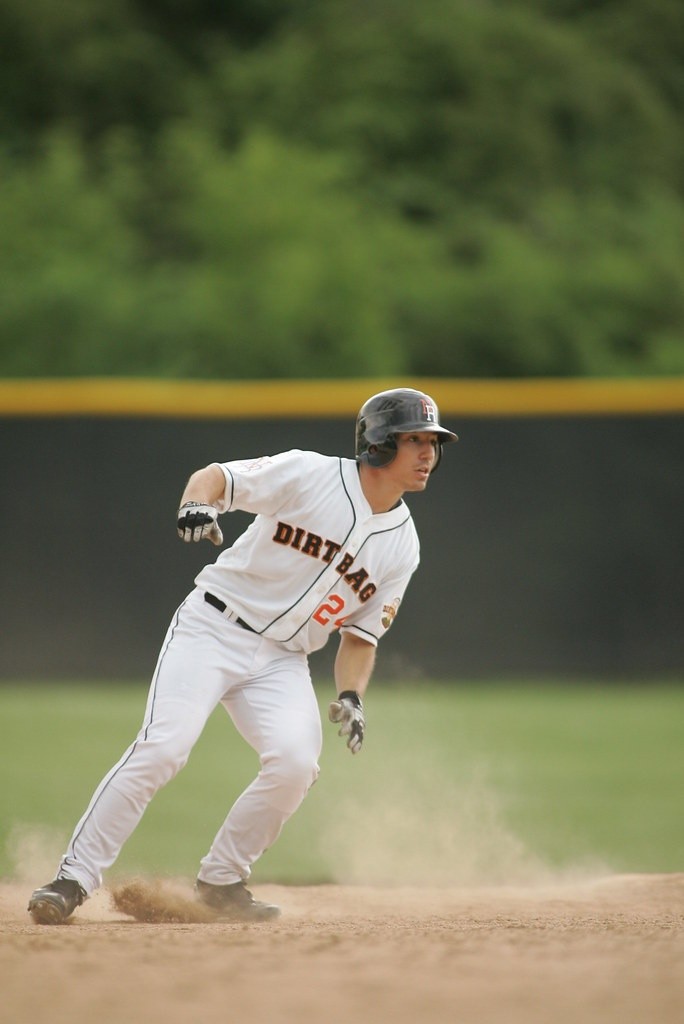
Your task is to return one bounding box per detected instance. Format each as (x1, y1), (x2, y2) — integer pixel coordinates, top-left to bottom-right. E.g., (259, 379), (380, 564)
(27, 389), (458, 926)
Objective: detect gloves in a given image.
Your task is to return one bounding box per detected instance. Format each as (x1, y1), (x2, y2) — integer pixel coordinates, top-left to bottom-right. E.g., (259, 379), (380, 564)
(328, 690), (365, 755)
(174, 502), (224, 548)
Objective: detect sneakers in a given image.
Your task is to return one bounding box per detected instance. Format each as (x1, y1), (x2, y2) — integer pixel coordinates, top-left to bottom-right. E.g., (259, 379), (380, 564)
(190, 876), (281, 919)
(30, 875), (89, 927)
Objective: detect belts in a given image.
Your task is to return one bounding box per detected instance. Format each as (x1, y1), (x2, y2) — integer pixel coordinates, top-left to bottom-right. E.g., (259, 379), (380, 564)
(199, 589), (269, 638)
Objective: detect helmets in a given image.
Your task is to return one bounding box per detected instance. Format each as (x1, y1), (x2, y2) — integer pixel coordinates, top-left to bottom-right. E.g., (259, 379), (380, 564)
(353, 387), (460, 475)
